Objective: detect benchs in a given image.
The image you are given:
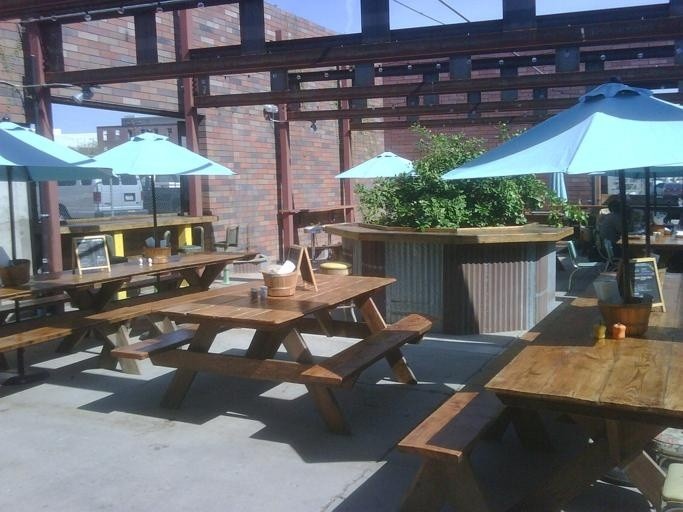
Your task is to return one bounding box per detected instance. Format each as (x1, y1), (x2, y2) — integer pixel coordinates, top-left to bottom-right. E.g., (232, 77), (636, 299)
(0, 265), (264, 381)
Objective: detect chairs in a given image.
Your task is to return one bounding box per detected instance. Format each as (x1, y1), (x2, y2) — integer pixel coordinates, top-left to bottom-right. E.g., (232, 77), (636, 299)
(162, 226), (239, 256)
(566, 224), (620, 294)
(105, 234), (128, 264)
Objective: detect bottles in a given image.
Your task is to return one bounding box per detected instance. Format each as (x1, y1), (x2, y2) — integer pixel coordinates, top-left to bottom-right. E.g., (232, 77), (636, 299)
(250, 286), (268, 301)
(138, 257), (143, 265)
(147, 257), (152, 266)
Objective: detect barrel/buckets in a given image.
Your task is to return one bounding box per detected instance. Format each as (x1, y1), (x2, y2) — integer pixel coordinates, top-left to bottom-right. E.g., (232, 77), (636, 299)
(0, 259), (30, 287)
(597, 294), (653, 335)
(261, 269), (298, 297)
(648, 223), (674, 234)
(143, 247), (171, 264)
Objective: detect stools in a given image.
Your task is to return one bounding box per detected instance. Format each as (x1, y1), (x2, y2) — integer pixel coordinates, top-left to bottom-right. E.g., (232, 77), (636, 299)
(276, 204), (356, 263)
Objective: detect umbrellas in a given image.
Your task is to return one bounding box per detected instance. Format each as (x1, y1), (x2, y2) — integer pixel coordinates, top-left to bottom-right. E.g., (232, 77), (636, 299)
(89, 127), (234, 295)
(590, 167), (682, 257)
(436, 81), (682, 295)
(0, 116), (116, 259)
(332, 150), (420, 180)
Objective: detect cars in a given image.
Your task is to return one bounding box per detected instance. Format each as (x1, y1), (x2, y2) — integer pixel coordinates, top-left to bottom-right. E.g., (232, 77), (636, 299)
(611, 175), (682, 195)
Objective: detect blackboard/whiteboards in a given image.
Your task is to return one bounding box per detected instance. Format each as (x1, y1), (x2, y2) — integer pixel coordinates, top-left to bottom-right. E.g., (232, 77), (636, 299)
(287, 247), (304, 274)
(72, 235), (110, 271)
(629, 257), (664, 307)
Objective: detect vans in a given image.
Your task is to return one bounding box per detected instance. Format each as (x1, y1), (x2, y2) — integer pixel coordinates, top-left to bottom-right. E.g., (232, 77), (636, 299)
(55, 164), (148, 227)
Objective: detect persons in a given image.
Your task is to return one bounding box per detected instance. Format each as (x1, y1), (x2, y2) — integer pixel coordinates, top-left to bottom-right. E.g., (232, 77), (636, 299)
(594, 194), (621, 247)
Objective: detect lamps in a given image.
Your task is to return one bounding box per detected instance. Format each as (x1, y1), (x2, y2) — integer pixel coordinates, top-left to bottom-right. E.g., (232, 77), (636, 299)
(71, 87), (93, 107)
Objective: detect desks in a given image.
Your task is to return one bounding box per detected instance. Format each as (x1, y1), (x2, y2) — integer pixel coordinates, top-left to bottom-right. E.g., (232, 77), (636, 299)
(616, 232), (682, 272)
(60, 213), (239, 256)
(34, 248), (260, 354)
(485, 273), (681, 512)
(150, 274), (417, 439)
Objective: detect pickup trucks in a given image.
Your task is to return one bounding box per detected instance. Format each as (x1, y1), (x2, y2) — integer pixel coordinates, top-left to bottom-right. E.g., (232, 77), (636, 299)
(139, 171), (180, 190)
